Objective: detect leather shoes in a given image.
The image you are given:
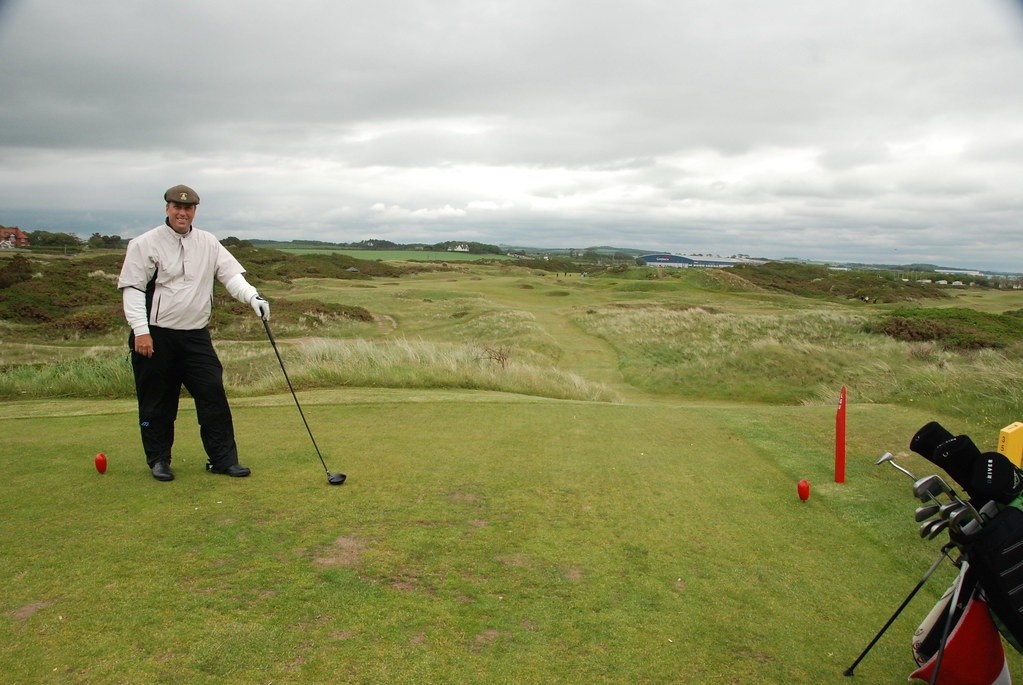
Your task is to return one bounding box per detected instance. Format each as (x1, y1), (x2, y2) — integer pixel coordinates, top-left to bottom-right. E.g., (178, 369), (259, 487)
(210, 463), (250, 477)
(152, 461), (174, 481)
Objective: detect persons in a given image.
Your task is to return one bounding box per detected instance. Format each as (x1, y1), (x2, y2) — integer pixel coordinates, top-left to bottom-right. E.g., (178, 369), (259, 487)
(117, 185), (270, 481)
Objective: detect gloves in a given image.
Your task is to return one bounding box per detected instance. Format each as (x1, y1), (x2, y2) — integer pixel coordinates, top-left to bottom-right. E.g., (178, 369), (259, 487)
(251, 295), (270, 322)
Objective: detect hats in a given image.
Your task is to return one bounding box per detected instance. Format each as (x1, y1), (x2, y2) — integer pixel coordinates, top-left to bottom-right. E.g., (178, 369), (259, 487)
(164, 185), (200, 205)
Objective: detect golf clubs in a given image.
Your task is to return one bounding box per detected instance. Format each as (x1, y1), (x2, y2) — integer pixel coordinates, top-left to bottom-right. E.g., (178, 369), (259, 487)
(259, 306), (347, 484)
(843, 452), (989, 685)
